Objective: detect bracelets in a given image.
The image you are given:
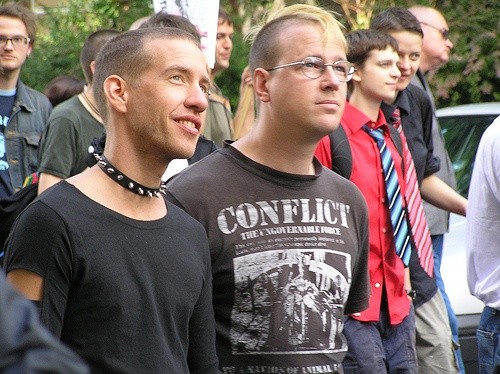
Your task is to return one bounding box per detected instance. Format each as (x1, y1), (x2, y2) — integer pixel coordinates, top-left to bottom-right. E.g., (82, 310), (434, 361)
(405, 289), (416, 300)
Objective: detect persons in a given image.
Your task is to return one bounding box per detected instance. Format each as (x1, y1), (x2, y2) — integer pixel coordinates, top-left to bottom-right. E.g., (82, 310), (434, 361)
(0, 1), (500, 374)
(3, 27), (221, 374)
(162, 4), (371, 373)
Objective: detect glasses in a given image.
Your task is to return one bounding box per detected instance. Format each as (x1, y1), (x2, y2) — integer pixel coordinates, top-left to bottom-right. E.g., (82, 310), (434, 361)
(0, 35), (30, 47)
(420, 22), (449, 40)
(265, 57), (358, 82)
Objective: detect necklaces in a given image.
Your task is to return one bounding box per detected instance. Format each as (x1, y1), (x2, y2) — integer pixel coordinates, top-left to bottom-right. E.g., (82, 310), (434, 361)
(92, 153), (168, 200)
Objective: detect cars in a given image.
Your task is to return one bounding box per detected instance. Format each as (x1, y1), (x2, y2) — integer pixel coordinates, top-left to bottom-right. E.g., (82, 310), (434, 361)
(409, 101), (499, 374)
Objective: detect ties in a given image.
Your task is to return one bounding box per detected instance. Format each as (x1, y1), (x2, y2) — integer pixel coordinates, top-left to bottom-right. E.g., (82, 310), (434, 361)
(391, 109), (434, 278)
(361, 126), (411, 266)
